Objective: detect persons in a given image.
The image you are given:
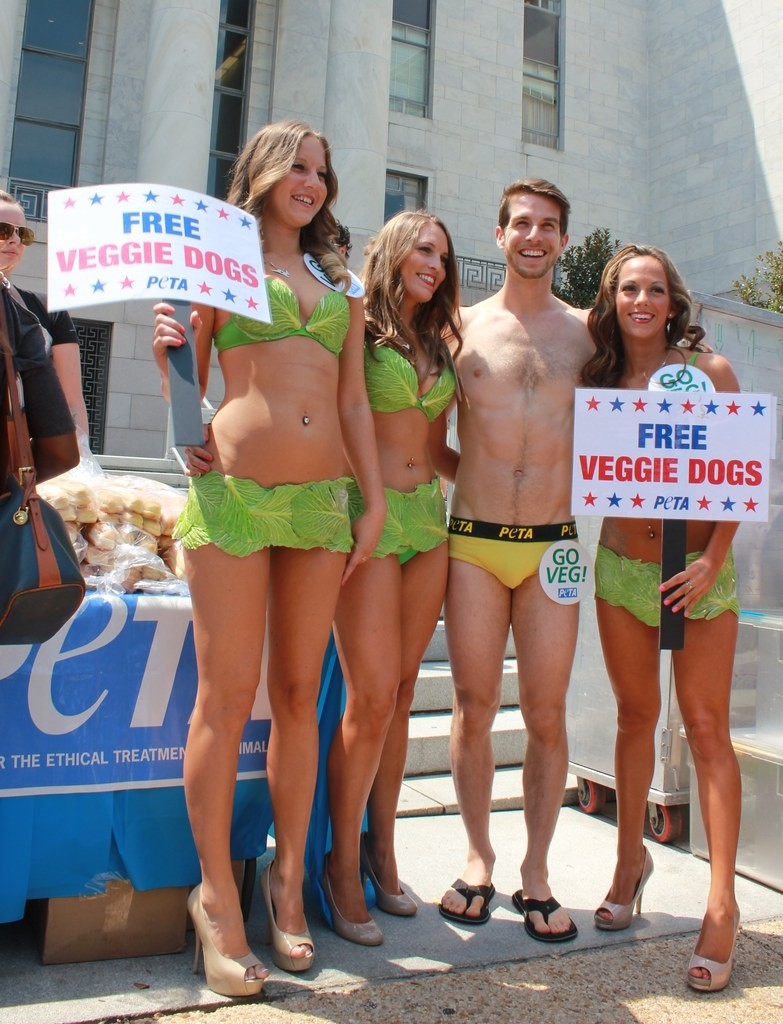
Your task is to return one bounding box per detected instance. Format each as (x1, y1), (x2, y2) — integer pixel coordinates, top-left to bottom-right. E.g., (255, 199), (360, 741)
(186, 207), (461, 946)
(151, 121), (389, 999)
(440, 175), (596, 942)
(580, 244), (744, 989)
(0, 190), (97, 507)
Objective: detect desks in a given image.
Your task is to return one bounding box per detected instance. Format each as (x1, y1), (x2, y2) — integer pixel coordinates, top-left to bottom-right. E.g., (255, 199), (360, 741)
(0, 580), (378, 929)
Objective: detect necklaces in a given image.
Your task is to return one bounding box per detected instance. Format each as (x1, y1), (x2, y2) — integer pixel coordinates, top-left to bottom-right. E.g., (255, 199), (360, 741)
(396, 335), (414, 353)
(264, 245), (304, 278)
(642, 345), (672, 382)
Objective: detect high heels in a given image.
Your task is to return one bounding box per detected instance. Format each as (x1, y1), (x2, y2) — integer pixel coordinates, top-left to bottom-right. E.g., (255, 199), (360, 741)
(187, 883), (264, 997)
(360, 831), (418, 916)
(260, 860), (314, 971)
(317, 852), (384, 946)
(687, 903), (740, 990)
(594, 845), (654, 930)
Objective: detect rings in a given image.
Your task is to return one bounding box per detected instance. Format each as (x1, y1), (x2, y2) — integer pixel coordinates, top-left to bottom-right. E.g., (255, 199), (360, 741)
(361, 556), (367, 563)
(685, 580), (695, 588)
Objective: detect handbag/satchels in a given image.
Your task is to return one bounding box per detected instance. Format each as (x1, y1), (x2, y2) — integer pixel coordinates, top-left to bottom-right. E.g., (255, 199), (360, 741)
(0, 472), (85, 645)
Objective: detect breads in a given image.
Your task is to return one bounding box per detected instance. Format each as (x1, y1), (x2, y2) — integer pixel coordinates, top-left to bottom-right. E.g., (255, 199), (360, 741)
(35, 478), (188, 594)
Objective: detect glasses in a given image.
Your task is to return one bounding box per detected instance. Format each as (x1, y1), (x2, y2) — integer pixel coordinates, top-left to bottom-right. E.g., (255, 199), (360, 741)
(0, 222), (35, 246)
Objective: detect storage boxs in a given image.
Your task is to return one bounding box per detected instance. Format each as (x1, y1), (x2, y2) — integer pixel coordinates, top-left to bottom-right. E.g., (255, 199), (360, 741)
(678, 726), (783, 895)
(33, 879), (189, 964)
(722, 607), (783, 757)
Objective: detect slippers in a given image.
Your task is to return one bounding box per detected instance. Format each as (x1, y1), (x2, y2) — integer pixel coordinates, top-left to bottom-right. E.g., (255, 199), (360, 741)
(512, 889), (578, 941)
(439, 879), (495, 923)
